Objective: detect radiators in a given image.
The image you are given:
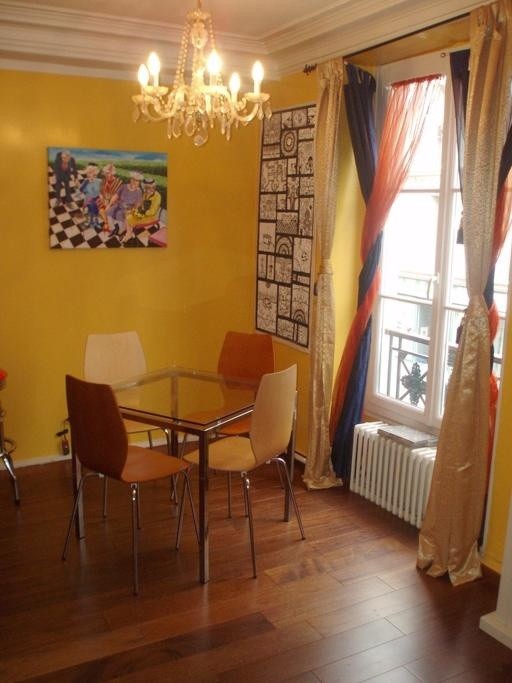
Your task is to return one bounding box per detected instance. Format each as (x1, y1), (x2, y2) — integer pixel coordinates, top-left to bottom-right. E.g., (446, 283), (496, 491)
(349, 420), (437, 530)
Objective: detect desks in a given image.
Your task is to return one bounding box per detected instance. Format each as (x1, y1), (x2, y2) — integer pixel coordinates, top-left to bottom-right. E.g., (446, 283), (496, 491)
(70, 365), (295, 584)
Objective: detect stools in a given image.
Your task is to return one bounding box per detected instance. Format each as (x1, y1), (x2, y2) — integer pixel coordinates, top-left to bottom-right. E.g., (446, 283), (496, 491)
(1, 367), (23, 504)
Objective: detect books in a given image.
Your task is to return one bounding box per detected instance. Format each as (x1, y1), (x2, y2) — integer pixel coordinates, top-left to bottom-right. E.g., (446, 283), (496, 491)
(378, 424), (438, 447)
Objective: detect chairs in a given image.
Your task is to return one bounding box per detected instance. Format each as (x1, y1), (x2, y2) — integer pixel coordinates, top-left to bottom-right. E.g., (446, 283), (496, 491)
(83, 331), (179, 517)
(61, 375), (200, 595)
(170, 331), (286, 517)
(176, 363), (306, 578)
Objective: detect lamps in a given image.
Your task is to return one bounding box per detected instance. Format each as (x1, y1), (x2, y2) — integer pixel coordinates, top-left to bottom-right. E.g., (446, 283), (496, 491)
(130, 0), (272, 146)
